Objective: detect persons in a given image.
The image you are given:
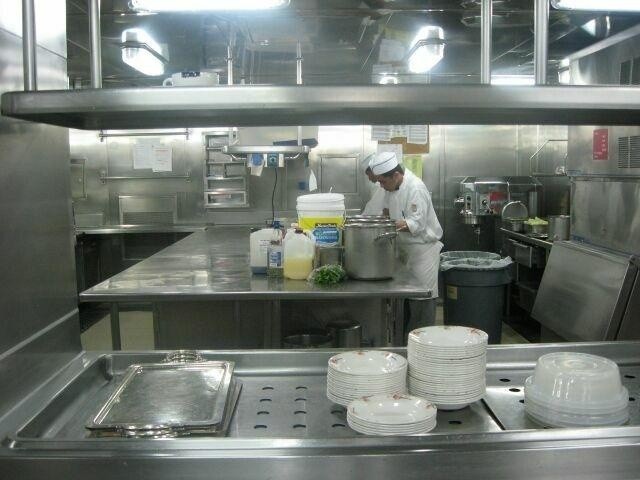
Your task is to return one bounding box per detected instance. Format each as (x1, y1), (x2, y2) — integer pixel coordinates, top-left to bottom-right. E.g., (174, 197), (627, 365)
(369, 151), (444, 339)
(361, 152), (389, 216)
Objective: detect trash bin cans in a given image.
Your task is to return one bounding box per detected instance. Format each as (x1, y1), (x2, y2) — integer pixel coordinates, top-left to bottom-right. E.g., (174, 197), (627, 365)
(441, 257), (512, 344)
(440, 251), (501, 325)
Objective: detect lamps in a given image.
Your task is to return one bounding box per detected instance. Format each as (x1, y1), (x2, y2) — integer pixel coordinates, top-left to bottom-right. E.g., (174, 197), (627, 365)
(405, 24), (445, 75)
(121, 28), (167, 76)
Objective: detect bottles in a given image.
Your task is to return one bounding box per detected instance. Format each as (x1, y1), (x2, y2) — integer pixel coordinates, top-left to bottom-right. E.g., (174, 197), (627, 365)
(267, 220), (284, 280)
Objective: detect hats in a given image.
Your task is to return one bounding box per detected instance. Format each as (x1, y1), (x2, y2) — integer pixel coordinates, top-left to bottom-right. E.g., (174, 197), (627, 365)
(360, 152), (376, 173)
(369, 152), (398, 175)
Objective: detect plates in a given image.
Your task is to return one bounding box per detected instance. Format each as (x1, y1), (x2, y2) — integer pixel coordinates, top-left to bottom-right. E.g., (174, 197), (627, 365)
(85, 349), (236, 431)
(89, 378), (246, 439)
(345, 394), (439, 435)
(406, 324), (490, 412)
(325, 349), (409, 407)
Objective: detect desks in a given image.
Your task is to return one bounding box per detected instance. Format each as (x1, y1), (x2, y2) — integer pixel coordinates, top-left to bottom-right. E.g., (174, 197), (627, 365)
(80, 225), (432, 351)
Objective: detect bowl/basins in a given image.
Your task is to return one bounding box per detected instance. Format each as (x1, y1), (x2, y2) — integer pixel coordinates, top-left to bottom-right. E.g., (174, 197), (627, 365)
(523, 351), (631, 429)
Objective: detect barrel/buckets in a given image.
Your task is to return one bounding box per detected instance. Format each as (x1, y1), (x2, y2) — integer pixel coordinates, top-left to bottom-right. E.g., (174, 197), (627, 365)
(295, 192), (347, 246)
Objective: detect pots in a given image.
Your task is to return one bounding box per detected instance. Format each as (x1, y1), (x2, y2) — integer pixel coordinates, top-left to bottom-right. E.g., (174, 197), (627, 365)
(347, 213), (389, 220)
(522, 216), (551, 236)
(339, 223), (400, 281)
(547, 214), (571, 242)
(344, 219), (397, 223)
(504, 215), (527, 233)
